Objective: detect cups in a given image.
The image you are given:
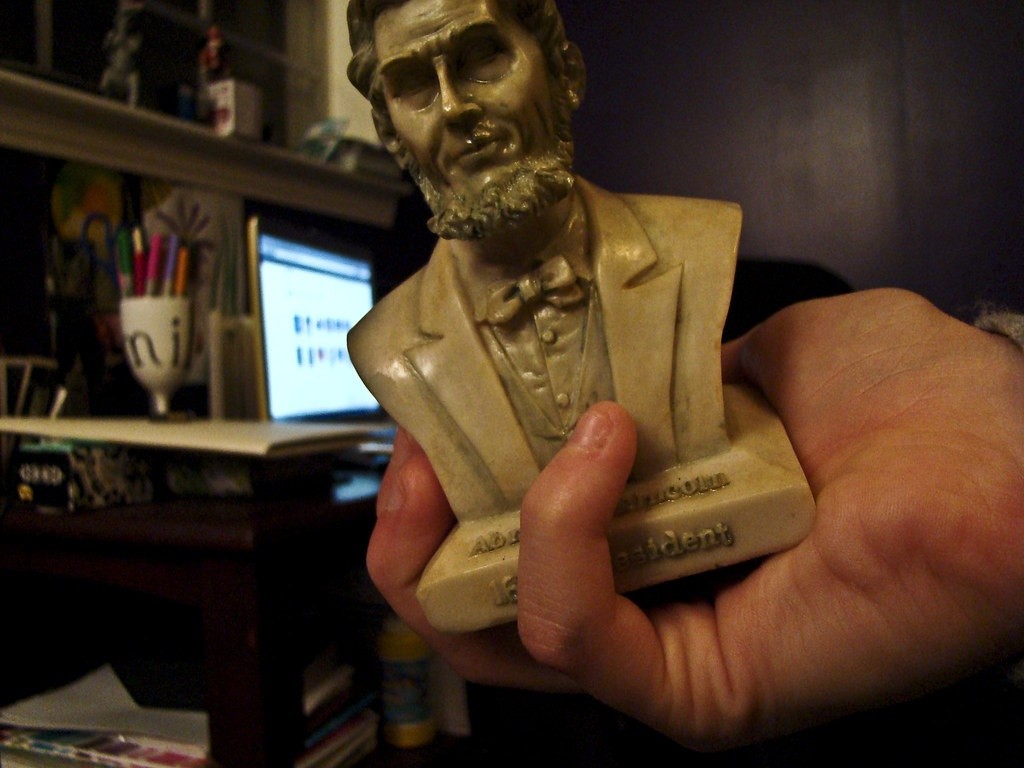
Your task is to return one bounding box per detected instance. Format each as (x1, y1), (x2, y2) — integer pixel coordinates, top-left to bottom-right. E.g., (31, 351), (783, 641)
(117, 293), (192, 416)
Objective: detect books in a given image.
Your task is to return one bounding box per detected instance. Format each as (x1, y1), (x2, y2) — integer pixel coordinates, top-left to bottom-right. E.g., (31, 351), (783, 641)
(0, 656), (377, 768)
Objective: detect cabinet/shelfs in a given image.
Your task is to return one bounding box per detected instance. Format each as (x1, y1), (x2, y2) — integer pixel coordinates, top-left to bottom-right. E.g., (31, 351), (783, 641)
(0, 0), (410, 415)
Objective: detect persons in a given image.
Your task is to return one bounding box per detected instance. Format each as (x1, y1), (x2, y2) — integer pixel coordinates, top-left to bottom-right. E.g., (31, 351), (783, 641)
(365, 289), (1023, 746)
(345, 1), (745, 519)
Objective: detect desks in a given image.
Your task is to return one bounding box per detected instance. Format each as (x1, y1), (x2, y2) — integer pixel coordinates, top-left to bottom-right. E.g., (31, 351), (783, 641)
(0, 462), (396, 768)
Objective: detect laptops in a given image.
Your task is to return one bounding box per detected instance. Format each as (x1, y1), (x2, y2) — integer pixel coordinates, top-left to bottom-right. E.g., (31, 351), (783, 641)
(248, 216), (399, 454)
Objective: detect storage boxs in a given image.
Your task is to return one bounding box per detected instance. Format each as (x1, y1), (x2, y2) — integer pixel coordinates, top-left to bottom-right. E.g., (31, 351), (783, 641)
(205, 79), (264, 143)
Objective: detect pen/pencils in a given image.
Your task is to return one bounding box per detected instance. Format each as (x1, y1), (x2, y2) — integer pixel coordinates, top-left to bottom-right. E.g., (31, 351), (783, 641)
(114, 224), (190, 299)
